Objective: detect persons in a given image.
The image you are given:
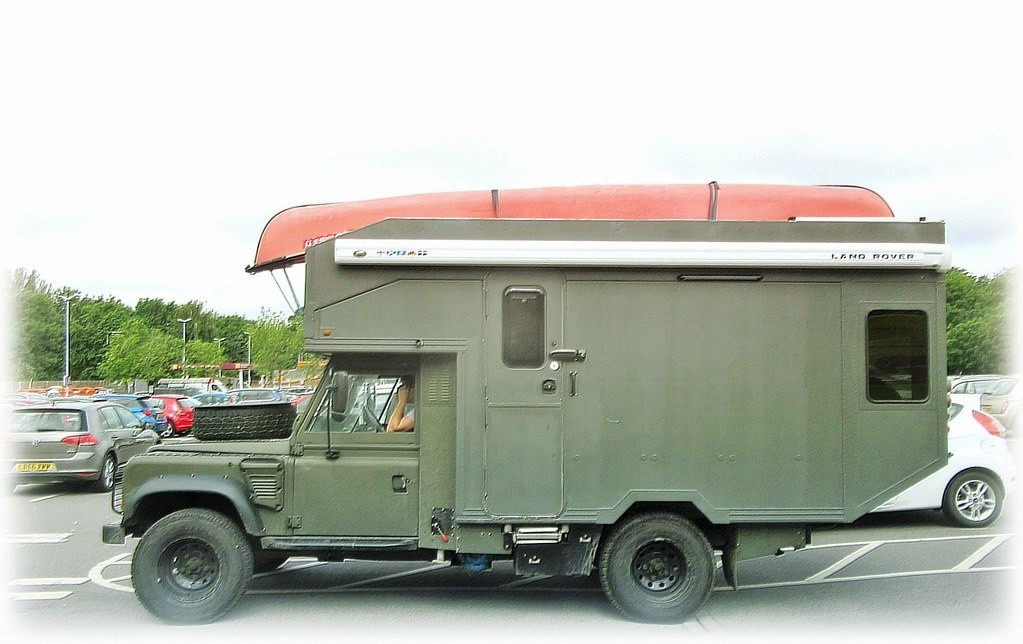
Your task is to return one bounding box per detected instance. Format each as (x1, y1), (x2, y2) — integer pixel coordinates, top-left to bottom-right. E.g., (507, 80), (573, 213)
(385, 375), (414, 433)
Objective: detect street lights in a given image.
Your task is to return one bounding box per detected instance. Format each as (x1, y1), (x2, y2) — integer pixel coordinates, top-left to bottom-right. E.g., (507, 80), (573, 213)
(58, 294), (80, 397)
(213, 338), (226, 377)
(178, 318), (192, 378)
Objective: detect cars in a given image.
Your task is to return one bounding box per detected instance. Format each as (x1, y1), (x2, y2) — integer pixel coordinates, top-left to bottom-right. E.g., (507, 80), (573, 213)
(947, 375), (1023, 439)
(4, 378), (313, 493)
(870, 396), (1014, 528)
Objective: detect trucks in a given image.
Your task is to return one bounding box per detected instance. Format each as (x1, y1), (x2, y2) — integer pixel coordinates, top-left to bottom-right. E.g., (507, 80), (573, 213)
(104, 216), (951, 626)
